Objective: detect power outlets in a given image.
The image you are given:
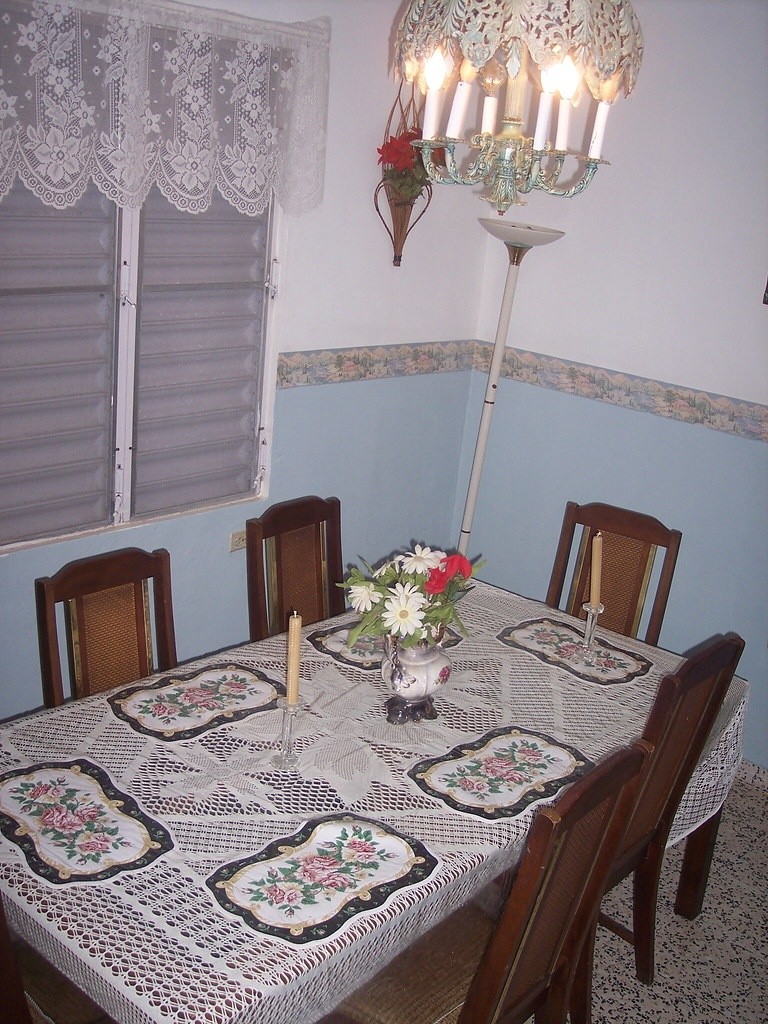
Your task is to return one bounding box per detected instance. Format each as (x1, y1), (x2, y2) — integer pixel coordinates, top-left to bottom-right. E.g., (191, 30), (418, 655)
(229, 530), (246, 552)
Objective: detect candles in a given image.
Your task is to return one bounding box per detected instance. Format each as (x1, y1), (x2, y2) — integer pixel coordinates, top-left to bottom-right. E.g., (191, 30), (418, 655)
(286, 610), (301, 706)
(590, 532), (602, 608)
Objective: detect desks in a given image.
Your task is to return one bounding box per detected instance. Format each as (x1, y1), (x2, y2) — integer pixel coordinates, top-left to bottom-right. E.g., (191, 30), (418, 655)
(1, 576), (751, 1024)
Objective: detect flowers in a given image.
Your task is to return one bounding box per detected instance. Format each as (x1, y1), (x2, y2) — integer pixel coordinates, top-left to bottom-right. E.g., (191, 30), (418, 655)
(377, 126), (447, 203)
(335, 544), (487, 650)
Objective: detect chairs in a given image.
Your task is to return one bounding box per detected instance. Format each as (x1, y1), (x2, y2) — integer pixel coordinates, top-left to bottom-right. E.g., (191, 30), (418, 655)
(567, 630), (745, 1024)
(35, 546), (177, 709)
(245, 495), (346, 642)
(335, 738), (656, 1024)
(544, 502), (682, 648)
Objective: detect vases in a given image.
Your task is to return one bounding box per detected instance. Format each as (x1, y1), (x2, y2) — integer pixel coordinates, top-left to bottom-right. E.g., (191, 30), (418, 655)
(381, 624), (452, 724)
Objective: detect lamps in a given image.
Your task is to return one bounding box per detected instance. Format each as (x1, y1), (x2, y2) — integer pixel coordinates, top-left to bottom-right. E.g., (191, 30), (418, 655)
(392, 0), (643, 216)
(457, 218), (566, 556)
